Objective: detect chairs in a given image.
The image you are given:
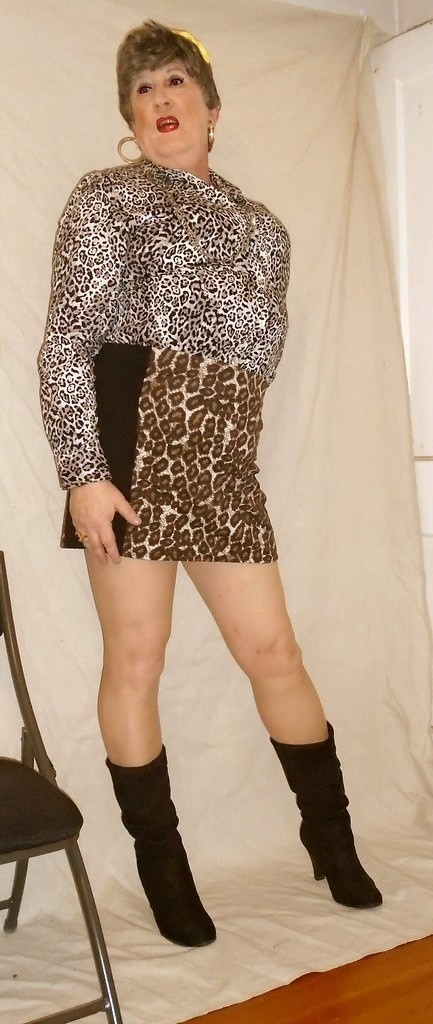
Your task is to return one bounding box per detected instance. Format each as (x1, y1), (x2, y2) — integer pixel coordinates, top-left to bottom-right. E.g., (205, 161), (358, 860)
(0, 551), (122, 1024)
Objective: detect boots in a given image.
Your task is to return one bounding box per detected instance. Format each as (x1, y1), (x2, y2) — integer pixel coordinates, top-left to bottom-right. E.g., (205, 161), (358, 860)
(270, 721), (384, 909)
(105, 744), (219, 946)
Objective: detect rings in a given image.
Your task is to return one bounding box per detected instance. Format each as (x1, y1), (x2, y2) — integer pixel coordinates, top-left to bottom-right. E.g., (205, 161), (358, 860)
(74, 532), (88, 542)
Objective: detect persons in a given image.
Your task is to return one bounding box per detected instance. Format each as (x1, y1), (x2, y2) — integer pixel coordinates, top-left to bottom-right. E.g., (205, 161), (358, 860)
(34, 21), (383, 947)
